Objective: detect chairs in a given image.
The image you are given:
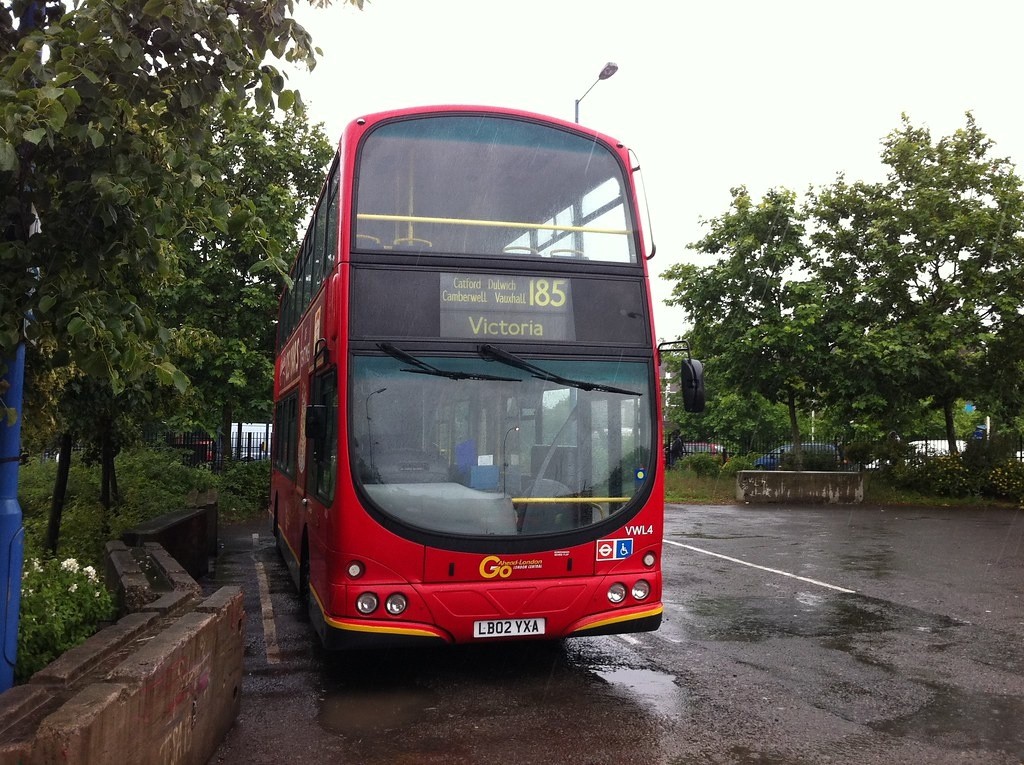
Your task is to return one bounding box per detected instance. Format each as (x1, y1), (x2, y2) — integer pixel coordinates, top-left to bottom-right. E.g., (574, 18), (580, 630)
(550, 249), (589, 260)
(355, 234), (384, 250)
(392, 237), (433, 251)
(500, 247), (542, 257)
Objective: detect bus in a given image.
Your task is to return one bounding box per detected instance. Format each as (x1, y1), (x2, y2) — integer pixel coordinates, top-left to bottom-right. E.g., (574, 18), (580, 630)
(270, 105), (706, 652)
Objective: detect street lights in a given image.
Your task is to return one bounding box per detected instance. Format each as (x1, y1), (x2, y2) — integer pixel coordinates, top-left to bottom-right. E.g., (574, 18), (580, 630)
(574, 61), (619, 255)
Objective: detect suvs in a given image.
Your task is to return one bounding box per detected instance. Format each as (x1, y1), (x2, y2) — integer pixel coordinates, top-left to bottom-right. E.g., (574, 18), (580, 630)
(663, 444), (729, 470)
(753, 443), (847, 470)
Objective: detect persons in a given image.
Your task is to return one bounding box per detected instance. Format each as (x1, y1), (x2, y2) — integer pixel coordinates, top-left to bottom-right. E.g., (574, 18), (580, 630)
(667, 429), (684, 470)
